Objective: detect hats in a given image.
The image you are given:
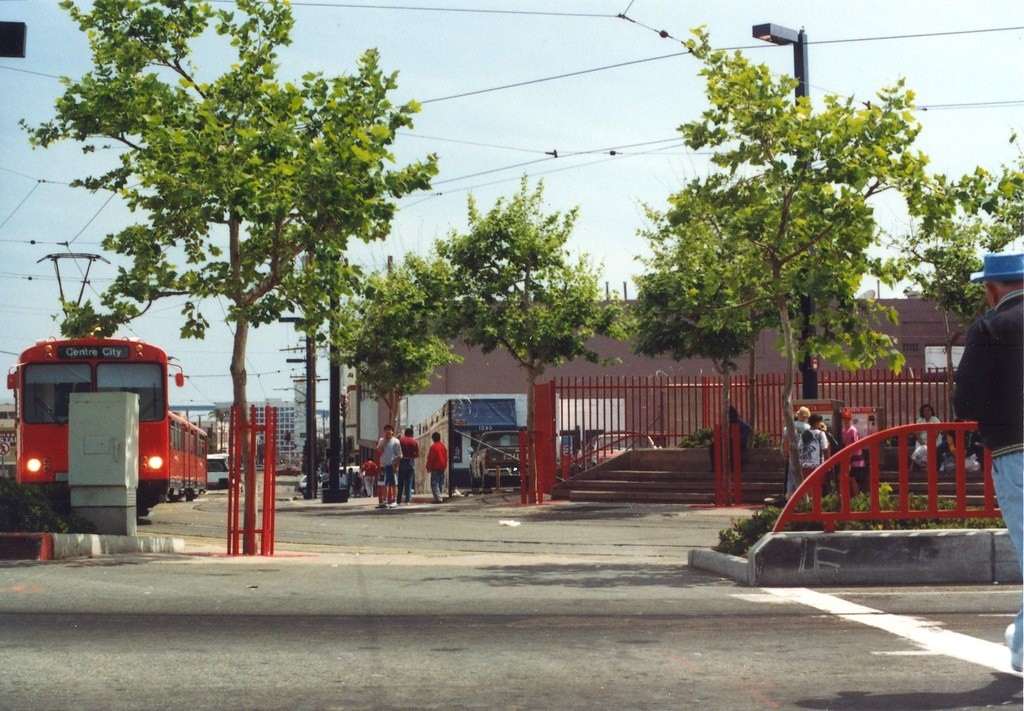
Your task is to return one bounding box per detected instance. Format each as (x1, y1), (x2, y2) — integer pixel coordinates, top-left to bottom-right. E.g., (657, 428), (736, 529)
(841, 411), (853, 418)
(818, 422), (827, 431)
(970, 253), (1024, 282)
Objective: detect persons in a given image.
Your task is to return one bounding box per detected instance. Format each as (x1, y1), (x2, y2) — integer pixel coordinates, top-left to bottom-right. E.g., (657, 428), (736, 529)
(706, 406), (753, 473)
(374, 424), (403, 509)
(317, 467), (367, 499)
(937, 417), (985, 474)
(398, 428), (420, 504)
(953, 251), (1024, 673)
(907, 404), (943, 473)
(425, 432), (448, 504)
(360, 456), (378, 498)
(781, 407), (866, 508)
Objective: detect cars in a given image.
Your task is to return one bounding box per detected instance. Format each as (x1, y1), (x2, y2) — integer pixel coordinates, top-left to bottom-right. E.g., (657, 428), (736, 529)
(295, 473), (325, 495)
(576, 433), (662, 474)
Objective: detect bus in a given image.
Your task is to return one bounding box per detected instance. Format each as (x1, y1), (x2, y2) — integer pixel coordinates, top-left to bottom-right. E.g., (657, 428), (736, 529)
(5, 337), (208, 518)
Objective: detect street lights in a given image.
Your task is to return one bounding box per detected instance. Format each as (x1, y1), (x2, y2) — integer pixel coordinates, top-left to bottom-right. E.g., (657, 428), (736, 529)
(751, 22), (820, 398)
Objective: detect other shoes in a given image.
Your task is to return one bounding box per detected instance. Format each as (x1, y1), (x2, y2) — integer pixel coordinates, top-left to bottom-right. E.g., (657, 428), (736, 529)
(379, 498), (387, 506)
(1005, 624), (1023, 671)
(389, 499), (398, 508)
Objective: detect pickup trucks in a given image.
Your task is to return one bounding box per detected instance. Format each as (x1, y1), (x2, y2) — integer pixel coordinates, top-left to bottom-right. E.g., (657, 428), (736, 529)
(467, 431), (529, 488)
